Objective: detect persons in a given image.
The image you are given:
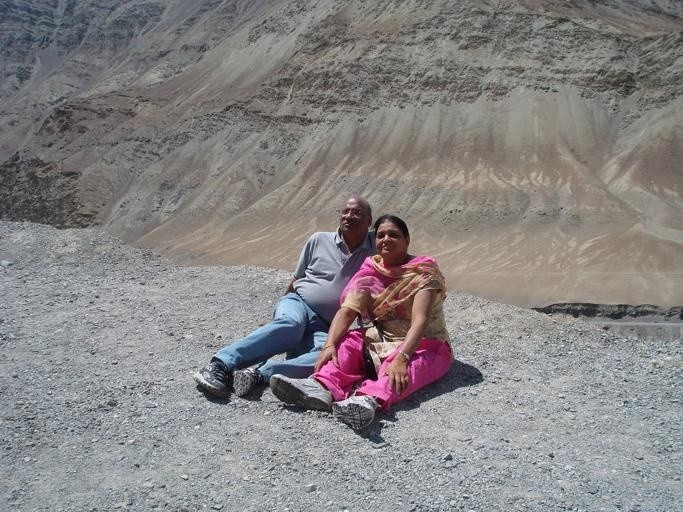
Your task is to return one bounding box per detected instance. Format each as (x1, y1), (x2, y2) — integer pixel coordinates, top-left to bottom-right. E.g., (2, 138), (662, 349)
(194, 195), (376, 399)
(269, 214), (454, 431)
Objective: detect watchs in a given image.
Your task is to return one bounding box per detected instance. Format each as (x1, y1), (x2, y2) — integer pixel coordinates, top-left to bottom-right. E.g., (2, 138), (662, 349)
(399, 350), (411, 361)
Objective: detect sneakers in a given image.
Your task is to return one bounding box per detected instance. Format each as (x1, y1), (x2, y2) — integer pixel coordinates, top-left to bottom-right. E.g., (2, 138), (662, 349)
(227, 368), (262, 396)
(331, 396), (382, 429)
(192, 361), (227, 396)
(270, 374), (333, 411)
